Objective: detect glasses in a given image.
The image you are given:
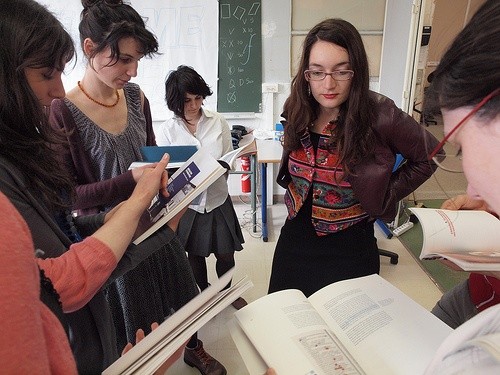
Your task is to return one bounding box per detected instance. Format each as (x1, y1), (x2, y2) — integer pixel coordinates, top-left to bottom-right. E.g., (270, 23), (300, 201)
(304, 69), (355, 82)
(426, 89), (500, 174)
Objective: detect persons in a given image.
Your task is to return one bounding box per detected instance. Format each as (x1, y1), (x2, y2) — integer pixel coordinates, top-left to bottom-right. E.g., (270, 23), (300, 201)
(265, 17), (446, 296)
(0, 0), (194, 375)
(426, 21), (500, 375)
(45, 0), (228, 375)
(152, 63), (248, 310)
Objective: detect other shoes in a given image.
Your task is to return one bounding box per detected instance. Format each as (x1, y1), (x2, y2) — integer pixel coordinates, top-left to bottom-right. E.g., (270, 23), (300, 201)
(232, 297), (247, 310)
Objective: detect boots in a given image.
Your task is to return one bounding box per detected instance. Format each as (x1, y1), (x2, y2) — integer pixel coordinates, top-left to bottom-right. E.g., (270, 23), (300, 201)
(183, 339), (227, 375)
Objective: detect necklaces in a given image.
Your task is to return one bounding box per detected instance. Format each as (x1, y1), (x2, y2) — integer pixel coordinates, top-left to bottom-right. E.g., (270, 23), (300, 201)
(77, 80), (119, 108)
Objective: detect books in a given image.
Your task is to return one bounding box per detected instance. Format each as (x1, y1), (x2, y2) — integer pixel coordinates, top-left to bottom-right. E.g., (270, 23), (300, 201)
(128, 137), (258, 170)
(407, 205), (500, 275)
(233, 272), (455, 375)
(101, 266), (253, 375)
(120, 146), (227, 245)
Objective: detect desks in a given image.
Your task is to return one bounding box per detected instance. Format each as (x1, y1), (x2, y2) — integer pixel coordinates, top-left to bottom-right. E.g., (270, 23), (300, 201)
(256, 140), (393, 242)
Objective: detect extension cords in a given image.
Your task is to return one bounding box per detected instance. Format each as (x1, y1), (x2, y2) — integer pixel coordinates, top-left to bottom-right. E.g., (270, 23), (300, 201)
(392, 222), (414, 237)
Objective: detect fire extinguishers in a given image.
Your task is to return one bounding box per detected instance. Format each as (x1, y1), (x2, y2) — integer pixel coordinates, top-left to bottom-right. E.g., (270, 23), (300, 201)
(241, 156), (251, 192)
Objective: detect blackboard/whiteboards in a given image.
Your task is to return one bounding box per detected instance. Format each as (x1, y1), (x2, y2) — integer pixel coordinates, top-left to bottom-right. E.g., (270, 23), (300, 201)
(218, 0), (261, 118)
